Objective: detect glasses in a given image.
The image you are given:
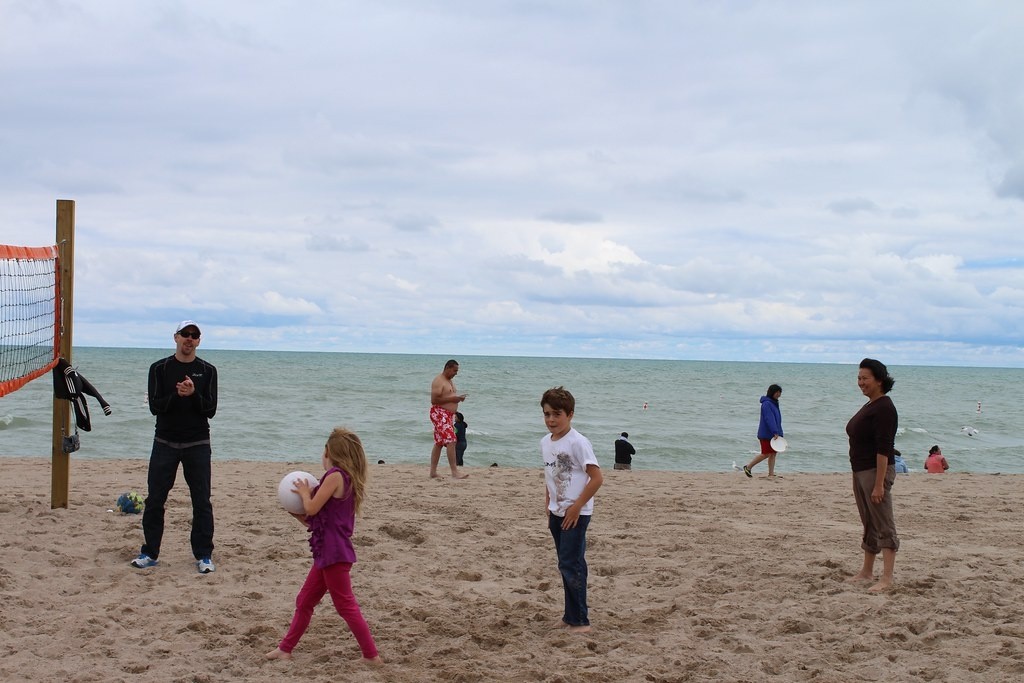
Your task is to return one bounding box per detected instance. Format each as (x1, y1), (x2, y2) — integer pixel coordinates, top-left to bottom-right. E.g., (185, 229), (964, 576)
(177, 331), (200, 339)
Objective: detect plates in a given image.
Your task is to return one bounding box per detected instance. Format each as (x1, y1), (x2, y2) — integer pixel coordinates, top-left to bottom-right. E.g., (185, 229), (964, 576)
(771, 436), (788, 452)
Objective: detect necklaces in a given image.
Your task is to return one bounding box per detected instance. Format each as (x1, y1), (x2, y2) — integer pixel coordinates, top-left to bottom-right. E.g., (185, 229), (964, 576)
(448, 380), (454, 392)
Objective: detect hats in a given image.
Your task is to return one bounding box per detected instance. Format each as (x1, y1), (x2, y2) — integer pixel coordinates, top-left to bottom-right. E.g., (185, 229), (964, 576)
(176, 320), (202, 335)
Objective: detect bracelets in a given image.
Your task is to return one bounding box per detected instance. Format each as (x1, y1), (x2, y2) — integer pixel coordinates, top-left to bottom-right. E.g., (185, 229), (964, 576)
(579, 494), (588, 501)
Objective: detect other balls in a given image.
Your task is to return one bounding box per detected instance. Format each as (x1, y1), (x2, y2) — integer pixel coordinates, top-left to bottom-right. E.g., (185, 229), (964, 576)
(117, 492), (144, 514)
(278, 471), (320, 515)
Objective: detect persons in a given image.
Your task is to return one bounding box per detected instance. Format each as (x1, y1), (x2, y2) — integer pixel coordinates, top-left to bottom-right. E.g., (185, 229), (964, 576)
(378, 460), (385, 464)
(643, 402), (648, 410)
(430, 360), (468, 478)
(924, 446), (949, 473)
(977, 403), (981, 411)
(743, 384), (783, 478)
(131, 320), (217, 573)
(454, 411), (468, 467)
(263, 427), (381, 665)
(540, 386), (602, 633)
(846, 358), (899, 592)
(894, 450), (908, 473)
(614, 432), (636, 470)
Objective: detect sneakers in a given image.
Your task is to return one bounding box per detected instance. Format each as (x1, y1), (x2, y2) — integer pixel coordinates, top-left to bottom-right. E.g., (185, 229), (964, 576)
(198, 558), (215, 573)
(743, 466), (753, 477)
(130, 553), (159, 569)
